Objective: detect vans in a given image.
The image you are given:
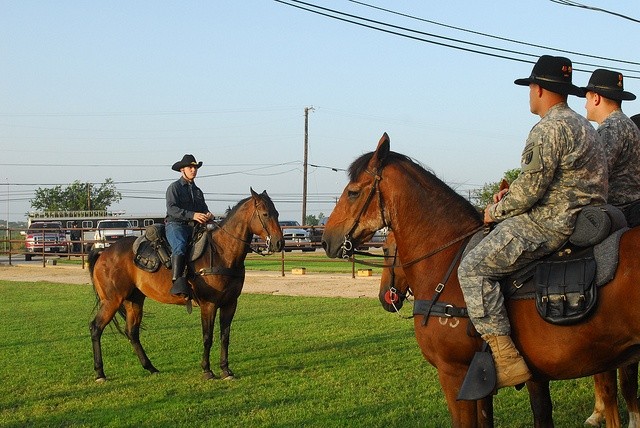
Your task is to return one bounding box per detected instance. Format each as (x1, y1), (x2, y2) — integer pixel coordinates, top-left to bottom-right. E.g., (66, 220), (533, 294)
(266, 228), (310, 252)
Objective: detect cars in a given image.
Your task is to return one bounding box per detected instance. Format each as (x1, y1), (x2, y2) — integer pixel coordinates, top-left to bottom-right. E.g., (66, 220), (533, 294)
(372, 231), (387, 247)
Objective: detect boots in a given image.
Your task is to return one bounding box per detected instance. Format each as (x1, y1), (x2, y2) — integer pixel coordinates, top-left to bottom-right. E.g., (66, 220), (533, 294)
(482, 333), (532, 392)
(172, 255), (186, 297)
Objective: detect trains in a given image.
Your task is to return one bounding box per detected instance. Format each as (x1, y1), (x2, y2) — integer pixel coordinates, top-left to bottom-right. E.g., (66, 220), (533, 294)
(27, 216), (315, 257)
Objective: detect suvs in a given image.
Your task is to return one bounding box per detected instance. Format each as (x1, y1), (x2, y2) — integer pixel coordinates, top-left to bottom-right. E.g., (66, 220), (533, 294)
(306, 227), (324, 251)
(91, 219), (134, 255)
(25, 221), (68, 261)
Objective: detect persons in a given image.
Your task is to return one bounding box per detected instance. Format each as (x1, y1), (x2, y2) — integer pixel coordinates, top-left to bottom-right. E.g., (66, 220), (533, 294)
(163, 155), (214, 295)
(574, 69), (640, 213)
(70, 221), (81, 257)
(456, 54), (609, 397)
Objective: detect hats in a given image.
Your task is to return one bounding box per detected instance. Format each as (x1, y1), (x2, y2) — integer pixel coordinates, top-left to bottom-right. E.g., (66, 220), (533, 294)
(514, 55), (578, 95)
(172, 154), (203, 172)
(578, 69), (636, 101)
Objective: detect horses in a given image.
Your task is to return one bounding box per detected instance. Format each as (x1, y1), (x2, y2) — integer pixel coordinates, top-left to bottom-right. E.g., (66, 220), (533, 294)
(87, 186), (285, 379)
(378, 227), (622, 426)
(321, 131), (640, 426)
